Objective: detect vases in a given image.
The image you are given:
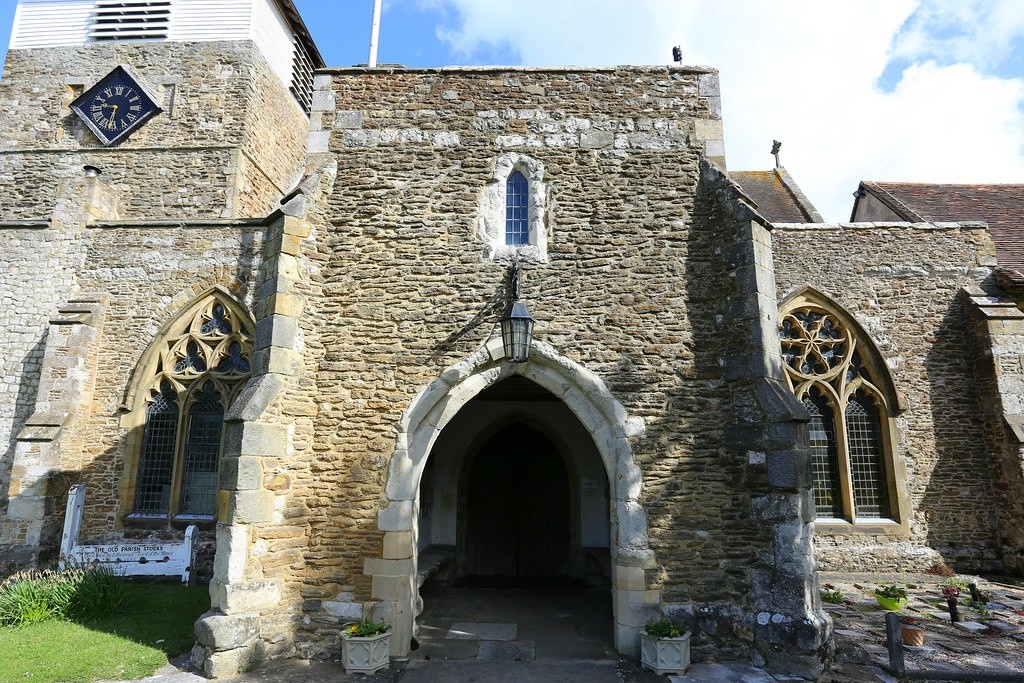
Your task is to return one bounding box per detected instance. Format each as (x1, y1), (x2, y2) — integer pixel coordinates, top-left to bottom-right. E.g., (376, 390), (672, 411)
(948, 601), (957, 624)
(902, 628), (925, 647)
(339, 629), (390, 675)
(640, 629), (692, 676)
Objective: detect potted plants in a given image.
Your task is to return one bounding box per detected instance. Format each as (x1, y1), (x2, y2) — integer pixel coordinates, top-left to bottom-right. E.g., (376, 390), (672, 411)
(968, 583), (984, 609)
(874, 586), (908, 611)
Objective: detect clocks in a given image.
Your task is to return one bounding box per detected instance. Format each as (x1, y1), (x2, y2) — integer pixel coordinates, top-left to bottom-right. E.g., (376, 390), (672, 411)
(69, 64), (163, 148)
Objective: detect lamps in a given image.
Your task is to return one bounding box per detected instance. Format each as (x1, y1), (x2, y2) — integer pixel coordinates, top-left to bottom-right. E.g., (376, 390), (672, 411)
(437, 261), (533, 362)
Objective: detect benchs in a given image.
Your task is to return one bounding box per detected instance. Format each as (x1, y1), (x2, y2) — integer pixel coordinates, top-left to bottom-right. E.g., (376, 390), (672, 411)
(416, 545), (455, 586)
(585, 547), (612, 583)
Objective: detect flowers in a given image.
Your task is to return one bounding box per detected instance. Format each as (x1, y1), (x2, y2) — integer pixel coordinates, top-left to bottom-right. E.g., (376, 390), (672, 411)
(645, 616), (687, 640)
(343, 618), (393, 638)
(899, 616), (923, 630)
(942, 586), (961, 602)
